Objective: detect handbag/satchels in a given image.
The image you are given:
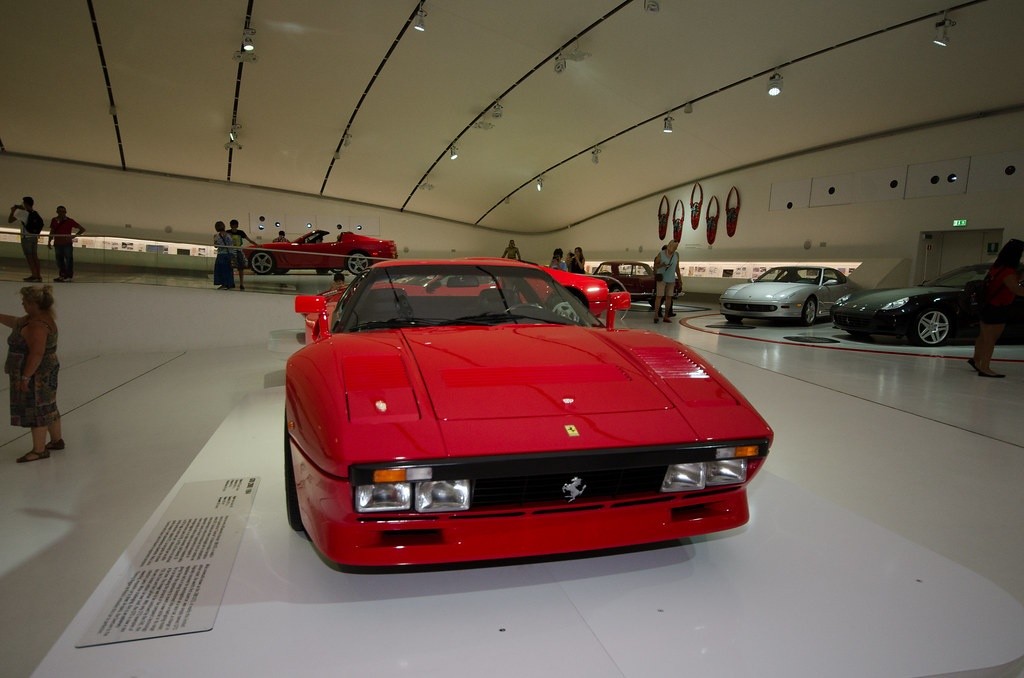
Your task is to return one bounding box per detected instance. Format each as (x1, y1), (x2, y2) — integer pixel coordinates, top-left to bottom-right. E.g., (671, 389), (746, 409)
(655, 273), (663, 281)
(229, 252), (240, 270)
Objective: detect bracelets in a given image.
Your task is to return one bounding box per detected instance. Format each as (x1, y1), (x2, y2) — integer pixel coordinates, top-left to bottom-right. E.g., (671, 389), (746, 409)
(22, 376), (31, 381)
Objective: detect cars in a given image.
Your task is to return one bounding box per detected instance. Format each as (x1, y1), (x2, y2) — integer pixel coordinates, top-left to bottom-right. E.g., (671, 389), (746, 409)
(829, 262), (1024, 345)
(586, 261), (684, 311)
(720, 265), (852, 326)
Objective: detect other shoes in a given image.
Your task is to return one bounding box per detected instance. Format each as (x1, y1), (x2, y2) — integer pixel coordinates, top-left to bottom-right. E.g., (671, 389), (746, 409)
(53, 276), (73, 283)
(663, 317), (672, 323)
(653, 317), (659, 324)
(24, 274), (42, 283)
(667, 312), (676, 317)
(240, 284), (246, 290)
(217, 284), (229, 291)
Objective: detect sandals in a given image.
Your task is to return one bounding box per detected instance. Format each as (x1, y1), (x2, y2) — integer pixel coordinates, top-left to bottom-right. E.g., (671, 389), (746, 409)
(46, 439), (65, 451)
(16, 445), (50, 463)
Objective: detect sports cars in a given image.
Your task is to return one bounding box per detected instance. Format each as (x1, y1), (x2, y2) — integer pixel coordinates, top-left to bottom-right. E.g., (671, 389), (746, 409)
(285, 258), (775, 574)
(241, 229), (398, 276)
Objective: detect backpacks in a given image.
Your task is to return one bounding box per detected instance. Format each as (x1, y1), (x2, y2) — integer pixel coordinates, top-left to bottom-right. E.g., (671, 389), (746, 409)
(20, 209), (44, 236)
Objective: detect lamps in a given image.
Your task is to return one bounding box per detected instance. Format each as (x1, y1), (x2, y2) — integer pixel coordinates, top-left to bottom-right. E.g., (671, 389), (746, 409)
(505, 195), (512, 204)
(224, 141), (245, 150)
(242, 27), (256, 51)
(537, 173), (545, 192)
(644, 1), (661, 14)
(420, 182), (434, 190)
(664, 111), (675, 134)
(592, 145), (601, 164)
(765, 66), (785, 95)
(231, 51), (259, 64)
(450, 142), (460, 160)
(933, 11), (959, 48)
(231, 123), (242, 140)
(333, 151), (342, 160)
(555, 54), (567, 73)
(414, 3), (430, 33)
(684, 102), (693, 114)
(344, 128), (353, 147)
(474, 119), (496, 129)
(492, 100), (504, 119)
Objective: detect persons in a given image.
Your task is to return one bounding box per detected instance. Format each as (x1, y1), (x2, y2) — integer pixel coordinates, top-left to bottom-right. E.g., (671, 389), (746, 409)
(654, 245), (676, 317)
(565, 252), (574, 268)
(48, 206), (86, 282)
(569, 247), (585, 274)
(214, 221), (234, 289)
(653, 240), (683, 323)
(968, 239), (1024, 378)
(8, 196), (42, 281)
(0, 285), (65, 463)
(226, 220), (262, 292)
(273, 231), (289, 242)
(549, 248), (567, 271)
(501, 240), (521, 260)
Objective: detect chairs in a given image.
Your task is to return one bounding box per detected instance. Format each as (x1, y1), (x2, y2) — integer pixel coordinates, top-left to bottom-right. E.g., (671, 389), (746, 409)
(815, 275), (829, 285)
(780, 273), (802, 282)
(474, 288), (524, 324)
(355, 288), (416, 331)
